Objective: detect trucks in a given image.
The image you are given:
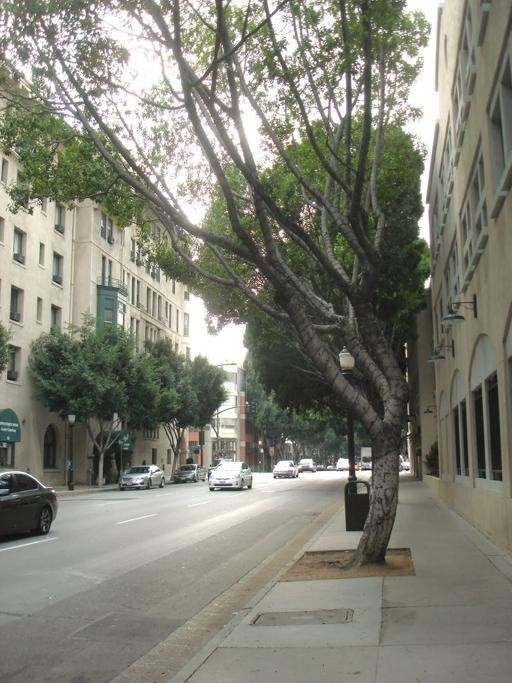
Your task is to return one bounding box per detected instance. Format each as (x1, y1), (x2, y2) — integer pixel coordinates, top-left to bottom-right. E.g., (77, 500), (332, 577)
(399, 454), (411, 471)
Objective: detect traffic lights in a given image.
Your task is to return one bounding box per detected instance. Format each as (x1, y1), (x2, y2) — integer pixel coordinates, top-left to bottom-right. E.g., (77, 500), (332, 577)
(252, 400), (257, 414)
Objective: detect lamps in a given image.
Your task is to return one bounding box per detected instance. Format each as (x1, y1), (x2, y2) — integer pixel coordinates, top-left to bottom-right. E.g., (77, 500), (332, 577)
(443, 294), (477, 324)
(428, 340), (454, 362)
(424, 405), (437, 415)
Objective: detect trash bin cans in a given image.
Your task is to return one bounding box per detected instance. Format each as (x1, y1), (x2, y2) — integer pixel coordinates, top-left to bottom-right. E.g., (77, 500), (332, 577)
(345, 481), (371, 531)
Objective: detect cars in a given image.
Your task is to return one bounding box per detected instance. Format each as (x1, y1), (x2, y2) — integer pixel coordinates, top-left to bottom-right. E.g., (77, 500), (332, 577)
(0, 468), (61, 538)
(172, 463), (206, 482)
(273, 457), (359, 477)
(119, 463), (165, 490)
(207, 457), (253, 490)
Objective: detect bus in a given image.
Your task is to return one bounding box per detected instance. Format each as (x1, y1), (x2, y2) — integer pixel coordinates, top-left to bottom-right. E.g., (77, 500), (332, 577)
(361, 456), (372, 469)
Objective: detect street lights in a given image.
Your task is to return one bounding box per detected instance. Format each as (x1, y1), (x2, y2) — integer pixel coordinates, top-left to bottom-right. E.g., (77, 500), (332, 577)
(339, 345), (356, 480)
(67, 413), (76, 490)
(119, 438), (124, 472)
(257, 440), (265, 472)
